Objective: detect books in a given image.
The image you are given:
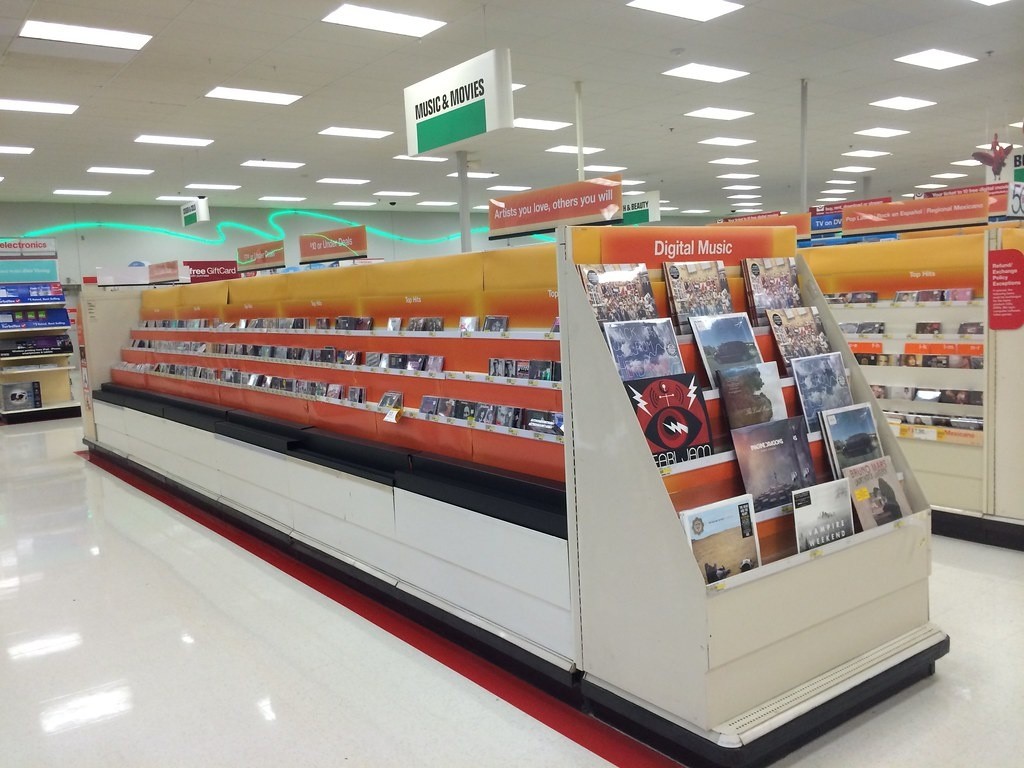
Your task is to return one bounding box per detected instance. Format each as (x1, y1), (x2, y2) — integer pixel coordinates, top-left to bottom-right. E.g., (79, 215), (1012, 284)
(577, 257), (913, 583)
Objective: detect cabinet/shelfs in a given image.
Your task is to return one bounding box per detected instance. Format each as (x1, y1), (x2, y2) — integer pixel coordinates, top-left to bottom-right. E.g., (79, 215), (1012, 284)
(0, 302), (82, 425)
(791, 233), (984, 542)
(564, 253), (931, 731)
(90, 287), (575, 685)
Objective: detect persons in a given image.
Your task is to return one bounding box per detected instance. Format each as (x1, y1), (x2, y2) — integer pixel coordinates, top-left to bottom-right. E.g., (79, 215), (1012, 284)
(118, 314), (561, 438)
(823, 289), (983, 430)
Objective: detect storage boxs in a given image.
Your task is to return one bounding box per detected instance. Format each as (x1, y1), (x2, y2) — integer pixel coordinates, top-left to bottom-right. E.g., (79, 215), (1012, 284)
(0, 381), (42, 411)
